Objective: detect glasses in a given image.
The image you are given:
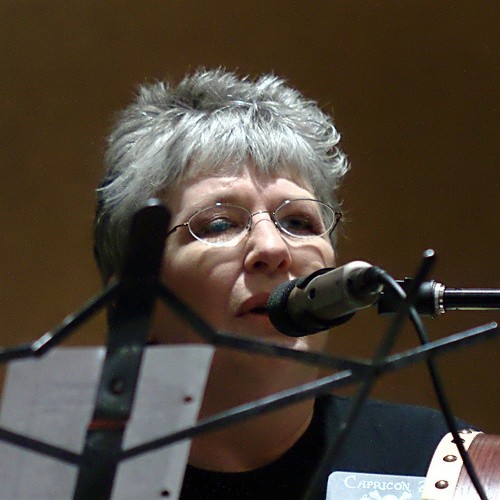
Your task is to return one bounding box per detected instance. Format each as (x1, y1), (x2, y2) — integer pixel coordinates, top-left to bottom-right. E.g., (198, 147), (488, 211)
(163, 198), (343, 248)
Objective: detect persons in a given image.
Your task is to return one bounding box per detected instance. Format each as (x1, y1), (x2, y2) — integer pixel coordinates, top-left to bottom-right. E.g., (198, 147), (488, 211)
(89, 63), (475, 500)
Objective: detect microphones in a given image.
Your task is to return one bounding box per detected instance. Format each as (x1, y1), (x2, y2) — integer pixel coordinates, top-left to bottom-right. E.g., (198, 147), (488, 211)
(378, 280), (500, 318)
(267, 261), (383, 337)
